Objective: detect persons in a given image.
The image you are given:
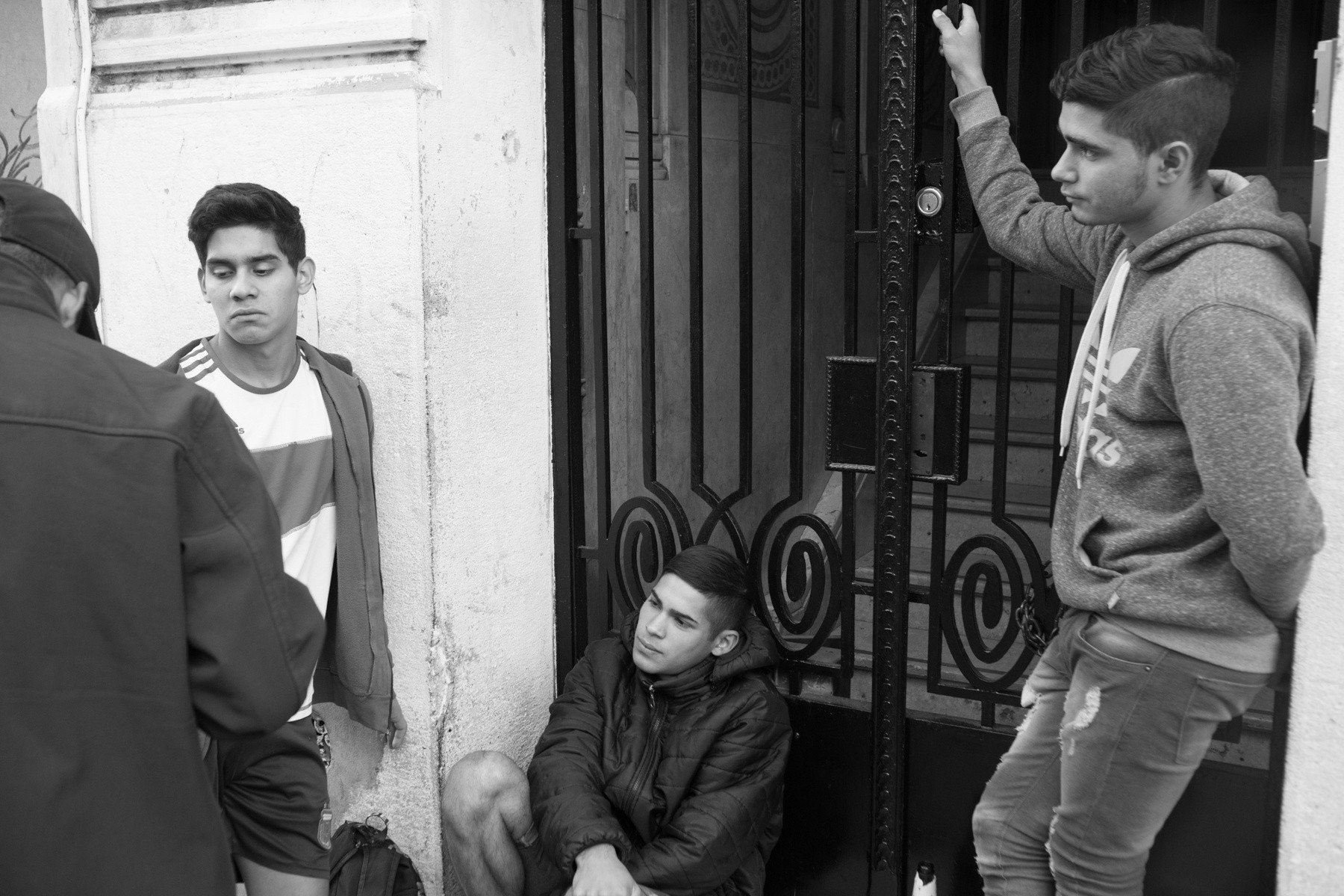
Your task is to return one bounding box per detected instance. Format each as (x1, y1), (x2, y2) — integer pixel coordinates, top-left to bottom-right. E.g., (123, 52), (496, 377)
(0, 180), (326, 896)
(150, 183), (405, 896)
(933, 0), (1326, 896)
(444, 545), (793, 896)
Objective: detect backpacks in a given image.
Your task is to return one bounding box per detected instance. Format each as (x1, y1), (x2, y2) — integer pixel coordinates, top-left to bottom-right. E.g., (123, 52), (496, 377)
(330, 820), (424, 896)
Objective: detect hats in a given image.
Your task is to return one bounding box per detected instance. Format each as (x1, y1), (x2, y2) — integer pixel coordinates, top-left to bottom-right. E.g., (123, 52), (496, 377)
(0, 178), (102, 346)
